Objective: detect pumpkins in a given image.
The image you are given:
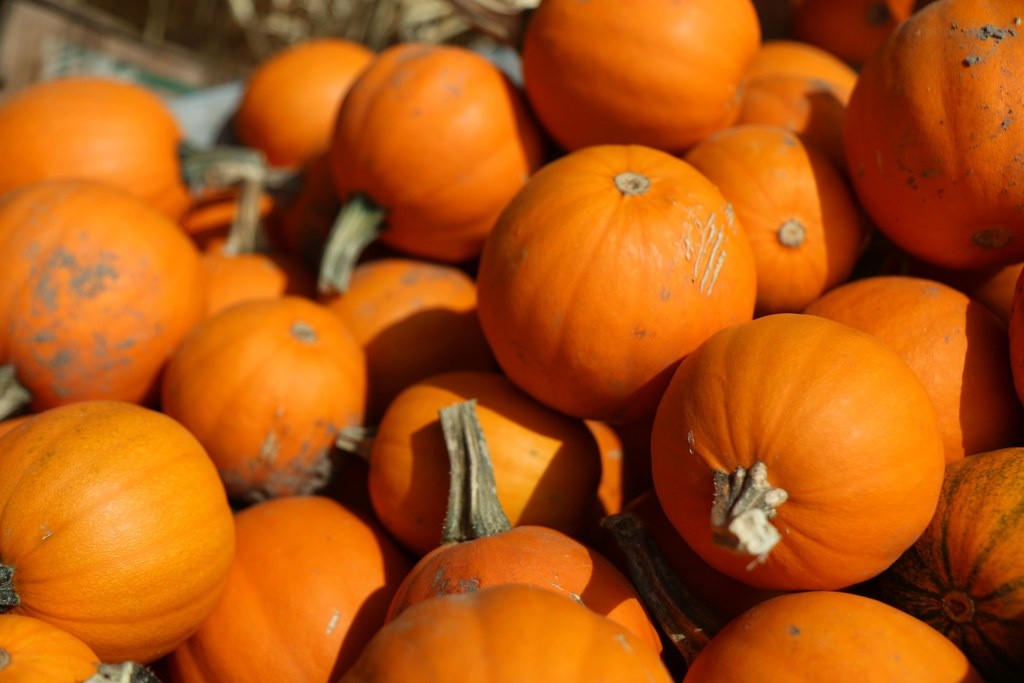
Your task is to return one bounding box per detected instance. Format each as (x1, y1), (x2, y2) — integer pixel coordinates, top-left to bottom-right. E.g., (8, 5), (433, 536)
(0, 0), (1024, 683)
